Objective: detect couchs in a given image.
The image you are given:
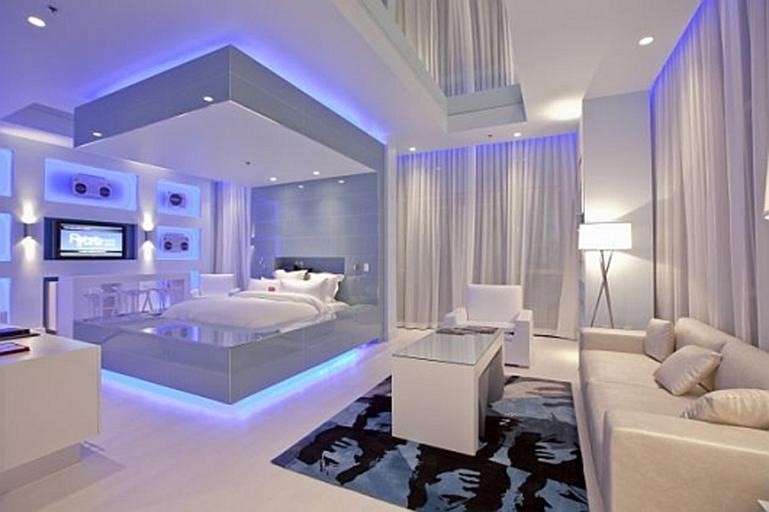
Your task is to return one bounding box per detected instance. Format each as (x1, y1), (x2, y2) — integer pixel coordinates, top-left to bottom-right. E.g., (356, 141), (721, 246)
(577, 314), (769, 512)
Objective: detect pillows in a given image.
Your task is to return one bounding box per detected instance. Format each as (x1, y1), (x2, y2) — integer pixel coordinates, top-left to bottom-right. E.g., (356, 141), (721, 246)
(678, 387), (769, 431)
(244, 265), (346, 305)
(644, 317), (675, 363)
(650, 341), (724, 396)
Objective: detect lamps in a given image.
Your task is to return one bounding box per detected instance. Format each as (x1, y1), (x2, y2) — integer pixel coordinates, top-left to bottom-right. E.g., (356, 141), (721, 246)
(576, 219), (633, 329)
(22, 222), (37, 238)
(145, 230), (156, 244)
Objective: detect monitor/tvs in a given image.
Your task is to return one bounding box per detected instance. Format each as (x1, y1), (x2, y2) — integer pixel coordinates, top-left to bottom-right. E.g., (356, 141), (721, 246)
(52, 221), (129, 260)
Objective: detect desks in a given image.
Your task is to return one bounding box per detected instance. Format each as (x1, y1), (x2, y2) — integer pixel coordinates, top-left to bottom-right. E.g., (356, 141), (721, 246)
(0, 320), (104, 499)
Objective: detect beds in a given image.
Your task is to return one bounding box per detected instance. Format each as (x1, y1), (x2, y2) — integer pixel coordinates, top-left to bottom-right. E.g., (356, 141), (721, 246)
(121, 290), (385, 407)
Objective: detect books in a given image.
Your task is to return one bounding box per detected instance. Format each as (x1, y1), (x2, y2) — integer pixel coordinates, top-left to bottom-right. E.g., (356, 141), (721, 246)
(0, 327), (39, 339)
(0, 342), (30, 354)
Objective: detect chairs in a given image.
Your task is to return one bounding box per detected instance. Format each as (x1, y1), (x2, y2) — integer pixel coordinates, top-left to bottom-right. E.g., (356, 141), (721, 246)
(442, 279), (535, 367)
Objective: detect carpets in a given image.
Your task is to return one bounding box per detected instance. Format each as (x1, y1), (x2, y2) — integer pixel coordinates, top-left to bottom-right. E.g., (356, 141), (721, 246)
(269, 370), (591, 512)
